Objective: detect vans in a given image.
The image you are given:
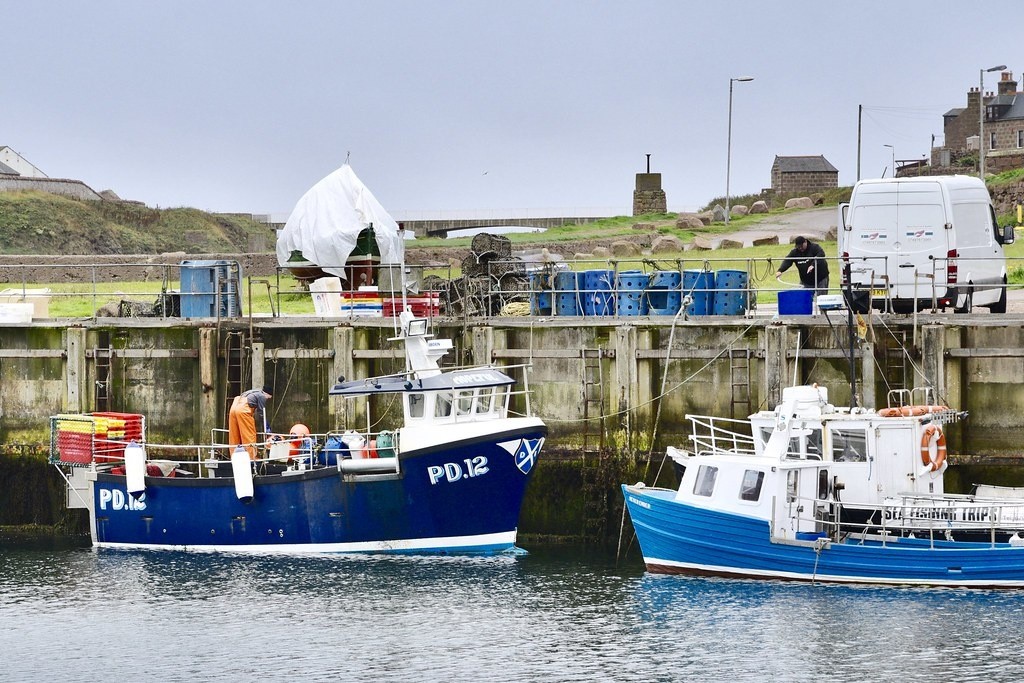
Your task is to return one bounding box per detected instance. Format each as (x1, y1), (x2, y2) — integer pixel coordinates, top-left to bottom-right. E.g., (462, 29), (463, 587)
(837, 174), (1015, 314)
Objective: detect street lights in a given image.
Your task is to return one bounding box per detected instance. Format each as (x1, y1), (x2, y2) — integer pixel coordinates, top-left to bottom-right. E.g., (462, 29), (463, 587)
(725, 75), (755, 225)
(979, 65), (1008, 186)
(884, 144), (894, 178)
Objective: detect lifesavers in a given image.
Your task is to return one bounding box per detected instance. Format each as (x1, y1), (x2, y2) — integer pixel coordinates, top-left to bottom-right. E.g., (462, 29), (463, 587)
(922, 426), (946, 471)
(878, 405), (949, 416)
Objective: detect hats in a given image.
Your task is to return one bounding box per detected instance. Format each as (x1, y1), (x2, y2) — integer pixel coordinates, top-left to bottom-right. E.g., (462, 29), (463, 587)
(795, 236), (806, 247)
(263, 386), (273, 395)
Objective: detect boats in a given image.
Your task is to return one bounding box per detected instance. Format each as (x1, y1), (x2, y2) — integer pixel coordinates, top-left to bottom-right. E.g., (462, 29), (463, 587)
(48, 220), (556, 561)
(621, 248), (1024, 592)
(280, 222), (381, 292)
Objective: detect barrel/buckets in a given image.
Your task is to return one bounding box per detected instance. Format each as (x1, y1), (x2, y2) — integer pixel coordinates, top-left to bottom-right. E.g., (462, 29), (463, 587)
(778, 290), (814, 316)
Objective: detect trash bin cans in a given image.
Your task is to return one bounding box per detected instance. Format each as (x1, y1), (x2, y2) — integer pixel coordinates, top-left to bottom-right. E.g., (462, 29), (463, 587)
(178, 260), (237, 319)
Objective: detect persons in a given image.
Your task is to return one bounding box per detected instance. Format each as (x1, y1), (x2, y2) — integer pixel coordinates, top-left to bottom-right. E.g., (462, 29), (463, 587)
(775, 236), (830, 315)
(229, 385), (273, 464)
